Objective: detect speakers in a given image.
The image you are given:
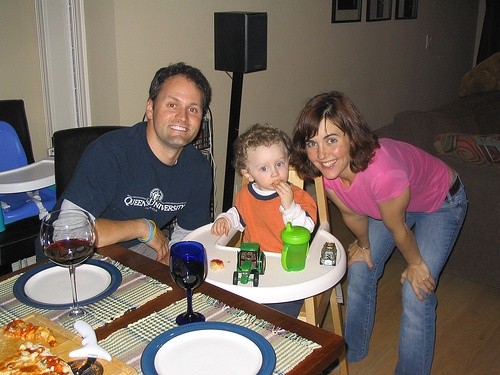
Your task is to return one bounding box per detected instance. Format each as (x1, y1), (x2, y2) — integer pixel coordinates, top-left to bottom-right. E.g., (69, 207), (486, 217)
(214, 11), (268, 74)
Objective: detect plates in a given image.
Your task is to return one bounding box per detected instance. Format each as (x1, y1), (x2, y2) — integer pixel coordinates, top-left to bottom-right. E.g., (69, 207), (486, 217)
(12, 259), (123, 310)
(40, 208), (101, 331)
(140, 321), (276, 375)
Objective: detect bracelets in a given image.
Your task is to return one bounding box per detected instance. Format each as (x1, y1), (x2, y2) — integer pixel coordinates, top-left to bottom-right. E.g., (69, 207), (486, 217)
(357, 238), (370, 250)
(137, 217), (156, 243)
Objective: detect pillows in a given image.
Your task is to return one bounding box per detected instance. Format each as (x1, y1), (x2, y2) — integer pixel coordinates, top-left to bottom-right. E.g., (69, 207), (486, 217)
(458, 52), (500, 98)
(434, 134), (500, 167)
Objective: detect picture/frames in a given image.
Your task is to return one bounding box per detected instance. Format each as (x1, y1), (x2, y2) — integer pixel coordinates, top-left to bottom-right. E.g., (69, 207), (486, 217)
(395, 0), (418, 20)
(366, 0), (392, 22)
(331, 0), (361, 22)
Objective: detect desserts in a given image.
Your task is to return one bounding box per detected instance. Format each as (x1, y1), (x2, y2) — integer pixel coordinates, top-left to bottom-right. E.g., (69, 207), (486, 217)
(273, 180), (282, 185)
(210, 259), (224, 269)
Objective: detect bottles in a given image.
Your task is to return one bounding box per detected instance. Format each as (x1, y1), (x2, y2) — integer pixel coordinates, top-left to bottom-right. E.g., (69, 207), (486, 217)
(281, 222), (312, 272)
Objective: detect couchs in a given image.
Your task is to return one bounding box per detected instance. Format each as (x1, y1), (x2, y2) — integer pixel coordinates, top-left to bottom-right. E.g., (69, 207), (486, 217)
(373, 89), (500, 289)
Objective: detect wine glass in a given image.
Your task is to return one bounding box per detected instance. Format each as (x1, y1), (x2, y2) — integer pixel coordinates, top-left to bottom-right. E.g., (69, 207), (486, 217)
(168, 241), (208, 323)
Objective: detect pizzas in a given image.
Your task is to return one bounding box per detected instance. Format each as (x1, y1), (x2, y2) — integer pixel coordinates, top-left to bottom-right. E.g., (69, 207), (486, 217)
(0, 341), (75, 375)
(2, 319), (57, 348)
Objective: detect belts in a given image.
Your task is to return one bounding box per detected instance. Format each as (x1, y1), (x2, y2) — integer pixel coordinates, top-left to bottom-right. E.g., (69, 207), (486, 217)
(444, 175), (460, 201)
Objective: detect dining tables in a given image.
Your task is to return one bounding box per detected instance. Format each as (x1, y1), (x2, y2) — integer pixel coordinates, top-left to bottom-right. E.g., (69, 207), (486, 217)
(0, 244), (347, 375)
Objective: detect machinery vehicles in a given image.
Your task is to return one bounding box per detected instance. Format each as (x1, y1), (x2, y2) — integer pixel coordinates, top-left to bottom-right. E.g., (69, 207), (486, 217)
(233, 242), (266, 287)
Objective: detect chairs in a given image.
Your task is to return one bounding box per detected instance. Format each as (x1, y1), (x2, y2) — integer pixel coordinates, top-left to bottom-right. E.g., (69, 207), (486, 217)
(52, 126), (131, 203)
(0, 121), (57, 232)
(182, 157), (348, 375)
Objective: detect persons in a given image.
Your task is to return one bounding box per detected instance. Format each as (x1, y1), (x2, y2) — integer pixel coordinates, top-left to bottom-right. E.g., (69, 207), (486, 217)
(34, 61), (214, 268)
(210, 122), (317, 320)
(287, 90), (468, 375)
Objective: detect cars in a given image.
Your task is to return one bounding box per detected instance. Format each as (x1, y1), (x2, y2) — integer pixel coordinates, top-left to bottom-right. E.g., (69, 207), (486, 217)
(320, 242), (337, 265)
